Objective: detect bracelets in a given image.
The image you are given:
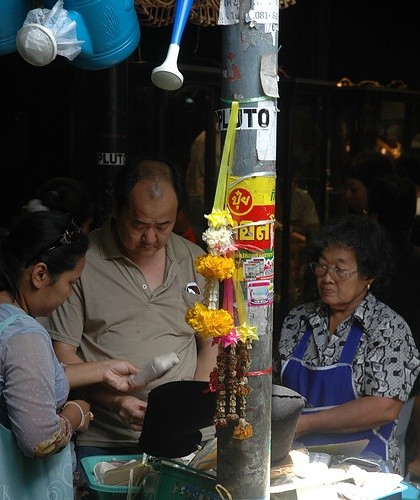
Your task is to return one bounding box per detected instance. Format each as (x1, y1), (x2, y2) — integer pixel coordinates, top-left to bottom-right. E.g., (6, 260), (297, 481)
(63, 401), (85, 430)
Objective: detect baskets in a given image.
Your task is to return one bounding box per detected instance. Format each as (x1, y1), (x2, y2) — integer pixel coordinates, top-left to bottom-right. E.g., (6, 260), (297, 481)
(146, 457), (218, 500)
(80, 454), (143, 500)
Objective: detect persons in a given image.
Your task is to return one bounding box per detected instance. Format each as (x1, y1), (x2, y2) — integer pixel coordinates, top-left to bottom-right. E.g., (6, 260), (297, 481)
(185, 121), (222, 211)
(0, 211), (91, 500)
(38, 177), (96, 234)
(338, 150), (420, 347)
(272, 225), (420, 477)
(290, 183), (320, 248)
(47, 160), (219, 480)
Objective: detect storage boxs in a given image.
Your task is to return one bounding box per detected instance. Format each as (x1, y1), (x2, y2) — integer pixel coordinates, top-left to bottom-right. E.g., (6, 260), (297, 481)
(82, 454), (146, 500)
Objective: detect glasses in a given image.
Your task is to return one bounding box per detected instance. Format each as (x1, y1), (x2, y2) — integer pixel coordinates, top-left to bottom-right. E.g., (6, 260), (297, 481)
(308, 257), (362, 283)
(186, 281), (201, 295)
(24, 216), (81, 271)
